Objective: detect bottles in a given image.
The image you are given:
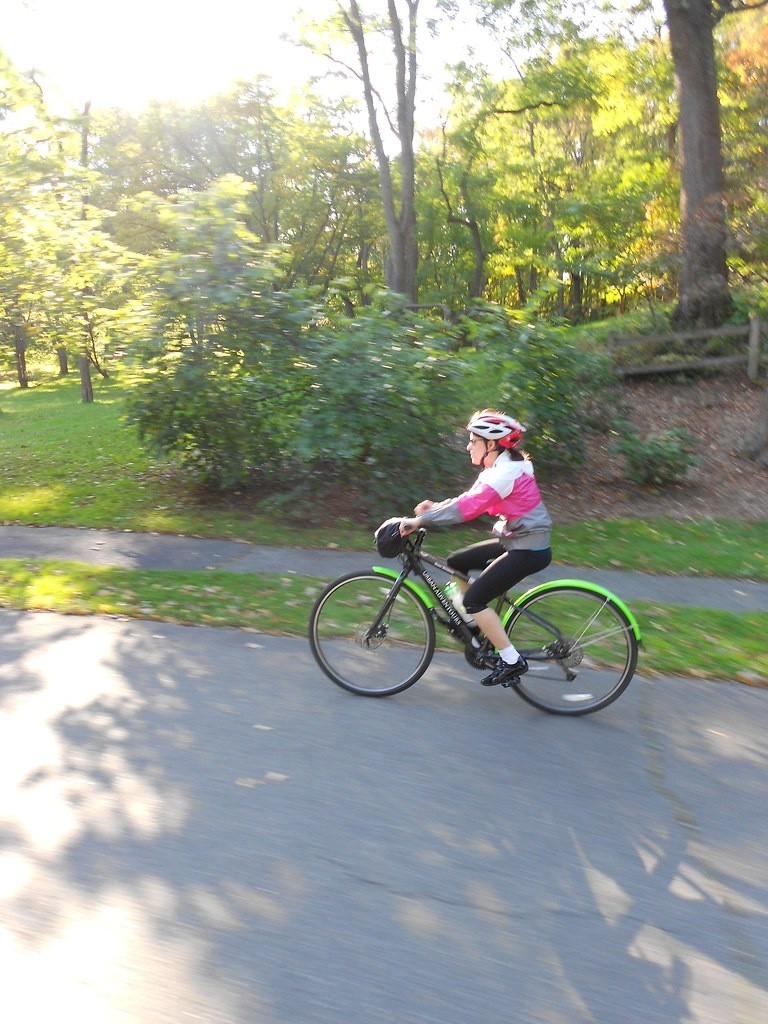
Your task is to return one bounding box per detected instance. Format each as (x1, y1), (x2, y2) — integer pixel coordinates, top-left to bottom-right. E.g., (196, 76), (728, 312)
(443, 581), (474, 624)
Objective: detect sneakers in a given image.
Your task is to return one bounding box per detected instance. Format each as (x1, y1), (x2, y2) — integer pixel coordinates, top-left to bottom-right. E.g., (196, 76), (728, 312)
(480, 655), (528, 686)
(439, 616), (480, 639)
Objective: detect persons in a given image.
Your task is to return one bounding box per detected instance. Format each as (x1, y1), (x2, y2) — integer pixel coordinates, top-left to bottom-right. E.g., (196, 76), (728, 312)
(400, 409), (552, 687)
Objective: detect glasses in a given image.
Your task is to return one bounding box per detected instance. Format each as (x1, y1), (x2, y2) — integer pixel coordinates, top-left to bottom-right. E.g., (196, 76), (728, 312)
(470, 438), (483, 444)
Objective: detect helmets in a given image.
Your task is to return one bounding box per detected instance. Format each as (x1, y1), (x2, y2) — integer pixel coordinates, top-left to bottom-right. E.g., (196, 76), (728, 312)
(466, 409), (527, 448)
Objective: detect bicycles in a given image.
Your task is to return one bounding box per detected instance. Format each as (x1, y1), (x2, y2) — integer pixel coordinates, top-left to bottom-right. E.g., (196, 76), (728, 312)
(310, 515), (643, 711)
